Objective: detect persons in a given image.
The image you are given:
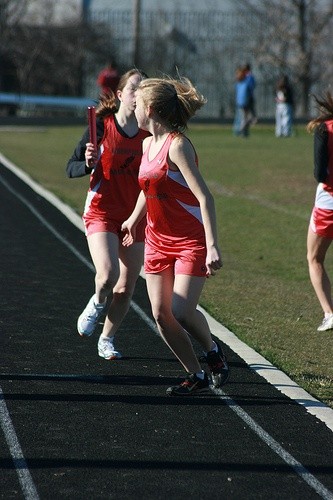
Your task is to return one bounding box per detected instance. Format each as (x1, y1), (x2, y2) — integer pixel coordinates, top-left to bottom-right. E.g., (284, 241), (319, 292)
(273, 75), (295, 137)
(306, 86), (333, 331)
(66, 60), (228, 393)
(234, 60), (257, 138)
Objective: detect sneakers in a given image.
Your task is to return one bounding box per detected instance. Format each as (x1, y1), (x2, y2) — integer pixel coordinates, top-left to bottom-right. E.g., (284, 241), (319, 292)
(198, 339), (231, 388)
(76, 293), (107, 338)
(169, 368), (210, 398)
(97, 333), (123, 361)
(316, 313), (333, 332)
